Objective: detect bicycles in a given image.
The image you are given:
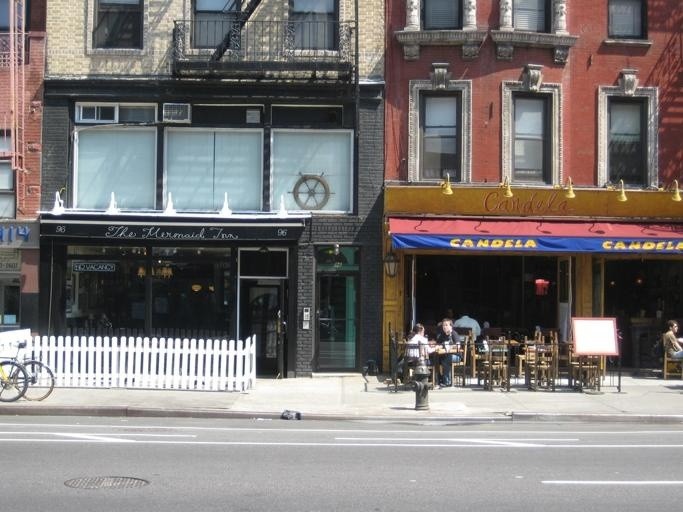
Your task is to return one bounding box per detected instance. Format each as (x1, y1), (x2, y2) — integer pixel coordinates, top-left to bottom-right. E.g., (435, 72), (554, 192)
(0, 339), (56, 403)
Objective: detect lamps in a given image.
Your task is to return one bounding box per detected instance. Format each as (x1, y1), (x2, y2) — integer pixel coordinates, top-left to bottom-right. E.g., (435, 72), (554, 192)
(163, 192), (177, 216)
(606, 179), (628, 202)
(50, 190), (65, 216)
(500, 175), (514, 197)
(136, 266), (173, 280)
(106, 192), (120, 217)
(658, 179), (682, 202)
(554, 176), (576, 198)
(441, 172), (454, 195)
(218, 191), (233, 217)
(275, 194), (289, 219)
(384, 243), (400, 279)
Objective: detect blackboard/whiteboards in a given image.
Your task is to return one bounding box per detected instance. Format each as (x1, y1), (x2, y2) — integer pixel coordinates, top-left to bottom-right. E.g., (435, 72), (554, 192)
(572, 317), (620, 356)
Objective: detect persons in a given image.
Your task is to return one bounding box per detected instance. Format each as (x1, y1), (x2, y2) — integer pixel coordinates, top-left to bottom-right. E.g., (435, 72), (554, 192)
(663, 319), (683, 372)
(399, 307), (481, 389)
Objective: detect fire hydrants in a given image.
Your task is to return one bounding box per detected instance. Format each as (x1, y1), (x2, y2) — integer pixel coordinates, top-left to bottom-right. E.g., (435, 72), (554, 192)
(410, 357), (432, 411)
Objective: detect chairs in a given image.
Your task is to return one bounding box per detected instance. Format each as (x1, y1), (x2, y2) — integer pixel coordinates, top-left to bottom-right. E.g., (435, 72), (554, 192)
(662, 333), (683, 381)
(389, 324), (607, 392)
